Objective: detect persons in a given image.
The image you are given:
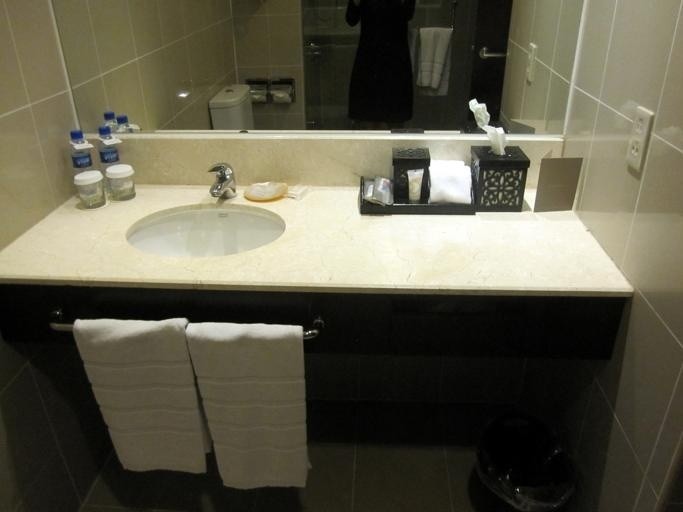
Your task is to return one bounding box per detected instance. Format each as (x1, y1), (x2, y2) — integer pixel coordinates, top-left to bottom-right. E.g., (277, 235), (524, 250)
(344, 0), (415, 130)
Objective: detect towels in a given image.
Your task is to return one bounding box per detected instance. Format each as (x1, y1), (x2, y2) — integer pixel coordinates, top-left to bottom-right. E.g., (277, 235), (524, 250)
(71, 318), (207, 477)
(416, 27), (453, 96)
(186, 316), (311, 489)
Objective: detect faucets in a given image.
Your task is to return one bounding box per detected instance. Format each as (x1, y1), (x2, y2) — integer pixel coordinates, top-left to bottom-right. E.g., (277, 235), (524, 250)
(205, 162), (236, 198)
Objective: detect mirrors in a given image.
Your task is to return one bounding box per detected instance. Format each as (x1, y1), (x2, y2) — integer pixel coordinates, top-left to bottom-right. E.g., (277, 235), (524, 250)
(50, 0), (586, 138)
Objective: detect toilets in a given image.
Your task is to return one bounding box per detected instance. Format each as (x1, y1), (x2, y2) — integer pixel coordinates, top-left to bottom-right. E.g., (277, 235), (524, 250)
(210, 83), (254, 130)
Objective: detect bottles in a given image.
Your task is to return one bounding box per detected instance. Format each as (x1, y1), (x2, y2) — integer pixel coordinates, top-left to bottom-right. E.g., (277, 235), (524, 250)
(73, 170), (106, 208)
(106, 165), (135, 201)
(68, 130), (95, 196)
(98, 127), (124, 193)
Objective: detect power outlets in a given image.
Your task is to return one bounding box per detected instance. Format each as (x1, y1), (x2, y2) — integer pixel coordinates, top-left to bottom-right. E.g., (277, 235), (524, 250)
(525, 42), (538, 83)
(625, 106), (654, 172)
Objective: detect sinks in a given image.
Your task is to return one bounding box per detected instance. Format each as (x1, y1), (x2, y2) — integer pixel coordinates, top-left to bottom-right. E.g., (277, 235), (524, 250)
(124, 204), (285, 258)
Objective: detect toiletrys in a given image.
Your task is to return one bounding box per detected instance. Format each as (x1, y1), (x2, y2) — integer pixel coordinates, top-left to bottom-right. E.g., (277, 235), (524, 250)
(407, 169), (424, 204)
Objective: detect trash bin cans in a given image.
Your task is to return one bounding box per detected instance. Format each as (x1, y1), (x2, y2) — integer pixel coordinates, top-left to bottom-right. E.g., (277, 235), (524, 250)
(468, 442), (580, 512)
